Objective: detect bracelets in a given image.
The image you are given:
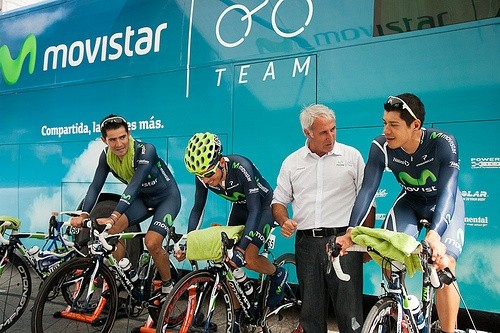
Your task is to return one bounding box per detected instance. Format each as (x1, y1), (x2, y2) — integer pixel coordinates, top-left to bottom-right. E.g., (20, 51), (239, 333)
(109, 214), (119, 225)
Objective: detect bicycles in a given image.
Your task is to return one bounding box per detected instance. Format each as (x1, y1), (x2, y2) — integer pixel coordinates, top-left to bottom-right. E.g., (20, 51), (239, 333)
(326, 219), (459, 333)
(0, 211), (202, 333)
(155, 224), (307, 333)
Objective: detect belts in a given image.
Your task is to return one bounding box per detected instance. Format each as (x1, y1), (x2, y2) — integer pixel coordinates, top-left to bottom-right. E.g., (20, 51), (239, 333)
(303, 226), (348, 238)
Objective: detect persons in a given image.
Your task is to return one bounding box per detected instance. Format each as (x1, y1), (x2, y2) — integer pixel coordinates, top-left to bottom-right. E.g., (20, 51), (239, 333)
(70, 113), (182, 306)
(325, 93), (465, 333)
(173, 132), (288, 310)
(270, 103), (375, 333)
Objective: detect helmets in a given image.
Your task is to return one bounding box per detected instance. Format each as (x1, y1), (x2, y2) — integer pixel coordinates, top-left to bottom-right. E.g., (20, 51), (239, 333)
(184, 132), (222, 174)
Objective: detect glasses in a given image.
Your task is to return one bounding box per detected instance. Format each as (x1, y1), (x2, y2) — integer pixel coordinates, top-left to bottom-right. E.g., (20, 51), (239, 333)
(100, 117), (128, 132)
(195, 161), (220, 179)
(385, 95), (418, 120)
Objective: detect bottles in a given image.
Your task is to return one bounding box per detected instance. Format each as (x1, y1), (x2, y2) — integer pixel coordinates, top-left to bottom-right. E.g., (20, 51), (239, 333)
(29, 245), (42, 260)
(408, 295), (426, 330)
(120, 258), (139, 282)
(233, 268), (254, 295)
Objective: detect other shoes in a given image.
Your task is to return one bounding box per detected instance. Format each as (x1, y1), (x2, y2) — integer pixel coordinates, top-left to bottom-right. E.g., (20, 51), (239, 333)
(160, 289), (171, 305)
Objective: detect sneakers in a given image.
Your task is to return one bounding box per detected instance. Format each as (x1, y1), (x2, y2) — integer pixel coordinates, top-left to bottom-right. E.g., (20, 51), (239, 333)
(266, 267), (289, 307)
(233, 308), (246, 333)
